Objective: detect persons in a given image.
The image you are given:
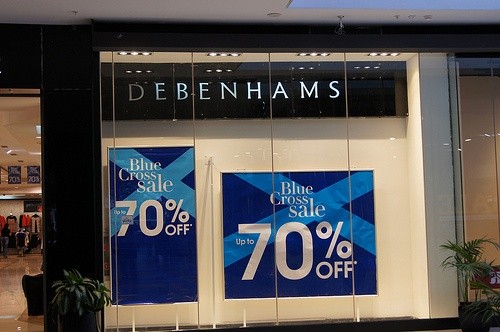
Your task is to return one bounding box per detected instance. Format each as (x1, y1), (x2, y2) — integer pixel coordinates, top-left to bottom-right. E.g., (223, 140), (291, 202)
(30, 213), (41, 234)
(1, 223), (11, 258)
(6, 213), (17, 236)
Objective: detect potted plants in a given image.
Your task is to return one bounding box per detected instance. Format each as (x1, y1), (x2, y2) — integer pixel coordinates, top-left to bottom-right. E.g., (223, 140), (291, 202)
(50, 269), (114, 332)
(439, 233), (500, 332)
